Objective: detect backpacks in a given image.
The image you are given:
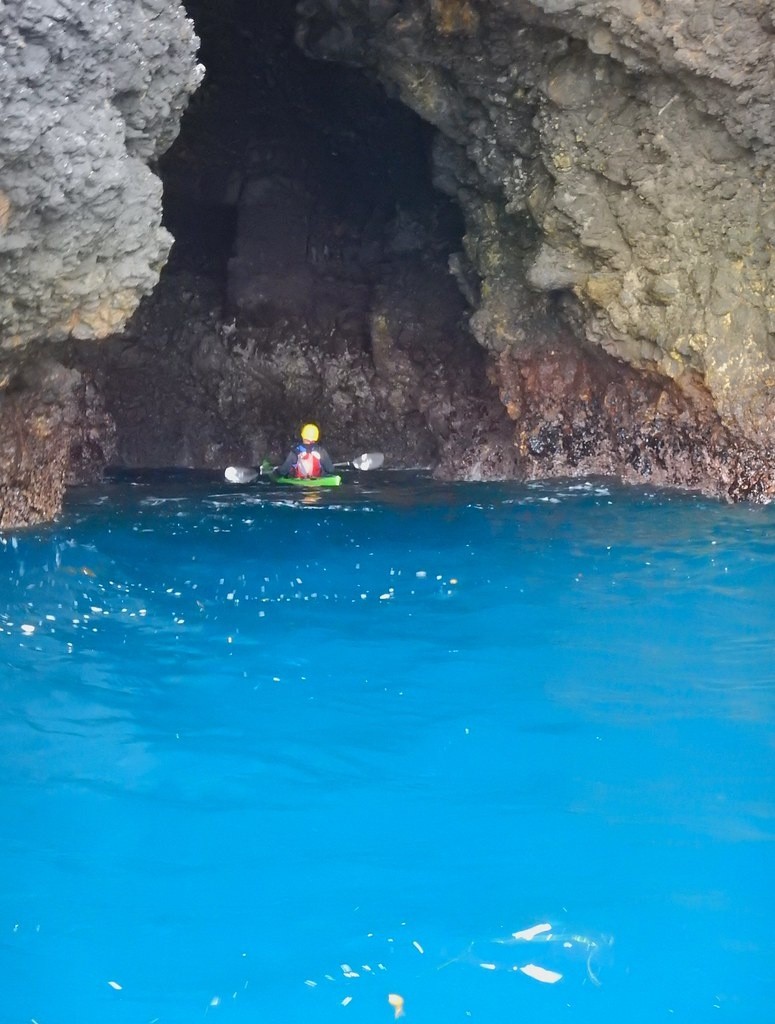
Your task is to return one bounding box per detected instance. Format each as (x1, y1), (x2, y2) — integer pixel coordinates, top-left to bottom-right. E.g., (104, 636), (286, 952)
(291, 447), (321, 477)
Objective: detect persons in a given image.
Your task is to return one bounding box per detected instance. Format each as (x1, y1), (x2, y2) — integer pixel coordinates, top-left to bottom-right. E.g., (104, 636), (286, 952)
(274, 423), (334, 480)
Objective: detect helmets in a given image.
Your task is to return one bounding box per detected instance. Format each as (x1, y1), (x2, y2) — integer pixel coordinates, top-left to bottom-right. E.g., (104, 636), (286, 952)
(301, 424), (319, 442)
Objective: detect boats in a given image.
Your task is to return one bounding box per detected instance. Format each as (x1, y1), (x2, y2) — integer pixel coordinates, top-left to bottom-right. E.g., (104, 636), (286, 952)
(263, 460), (340, 488)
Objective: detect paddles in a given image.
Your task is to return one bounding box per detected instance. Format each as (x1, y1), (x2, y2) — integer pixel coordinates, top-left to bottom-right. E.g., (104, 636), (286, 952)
(225, 452), (384, 484)
